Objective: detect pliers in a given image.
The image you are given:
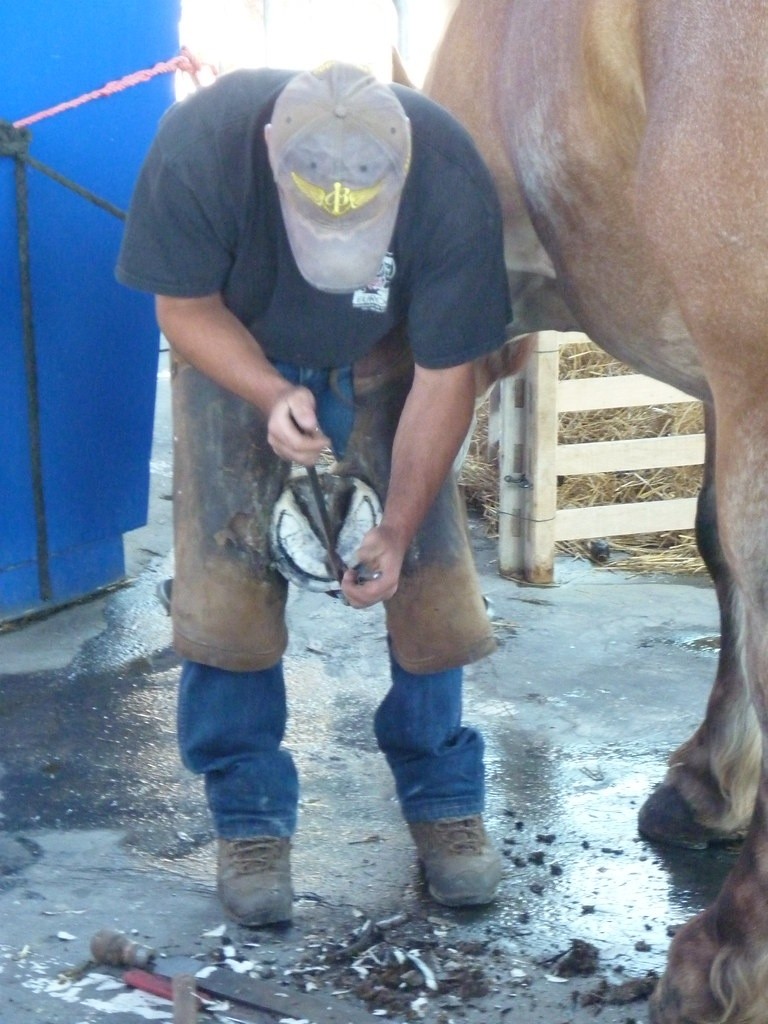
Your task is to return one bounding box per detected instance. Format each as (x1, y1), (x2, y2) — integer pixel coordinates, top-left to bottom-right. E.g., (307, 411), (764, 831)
(306, 465), (385, 582)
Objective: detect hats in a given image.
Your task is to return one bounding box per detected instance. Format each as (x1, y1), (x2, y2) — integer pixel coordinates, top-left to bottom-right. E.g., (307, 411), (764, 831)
(270, 64), (410, 293)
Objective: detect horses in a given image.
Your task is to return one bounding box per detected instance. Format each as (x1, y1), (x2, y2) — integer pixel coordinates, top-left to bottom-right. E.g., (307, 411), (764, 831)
(263, 0), (766, 1023)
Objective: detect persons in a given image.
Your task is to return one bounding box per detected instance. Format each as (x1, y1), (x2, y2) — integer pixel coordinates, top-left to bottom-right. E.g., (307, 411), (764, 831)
(117, 65), (512, 928)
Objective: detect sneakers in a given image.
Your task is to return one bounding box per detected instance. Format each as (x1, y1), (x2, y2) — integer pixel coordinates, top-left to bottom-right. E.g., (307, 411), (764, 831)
(407, 813), (500, 906)
(217, 834), (291, 923)
